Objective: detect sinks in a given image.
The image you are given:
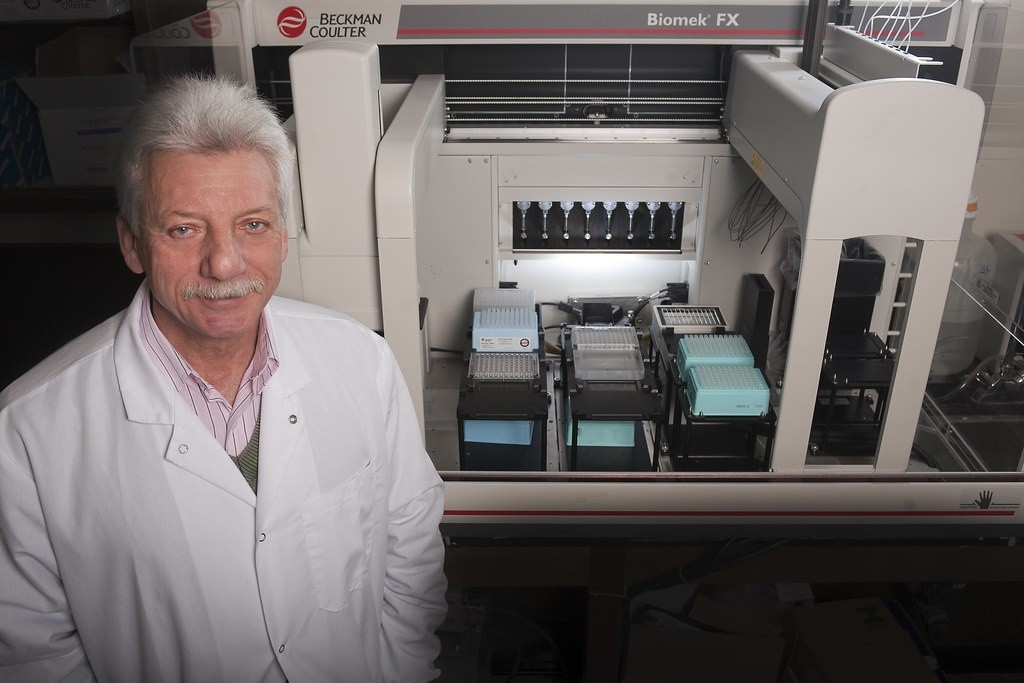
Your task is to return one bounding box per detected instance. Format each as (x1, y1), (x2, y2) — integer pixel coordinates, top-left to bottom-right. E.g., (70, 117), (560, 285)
(943, 421), (1024, 472)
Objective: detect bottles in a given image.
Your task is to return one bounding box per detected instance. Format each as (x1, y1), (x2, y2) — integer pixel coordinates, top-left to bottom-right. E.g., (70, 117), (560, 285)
(925, 196), (997, 386)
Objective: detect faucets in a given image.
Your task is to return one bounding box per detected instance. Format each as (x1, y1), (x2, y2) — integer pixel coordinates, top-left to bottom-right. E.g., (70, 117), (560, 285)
(959, 369), (1001, 391)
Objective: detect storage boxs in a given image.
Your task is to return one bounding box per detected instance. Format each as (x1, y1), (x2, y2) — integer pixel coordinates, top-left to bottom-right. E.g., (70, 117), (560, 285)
(0, 23), (149, 190)
(618, 570), (947, 683)
(434, 582), (493, 683)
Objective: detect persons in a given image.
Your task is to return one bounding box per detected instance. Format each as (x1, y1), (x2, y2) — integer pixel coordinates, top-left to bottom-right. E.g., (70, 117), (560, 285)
(0, 76), (448, 683)
